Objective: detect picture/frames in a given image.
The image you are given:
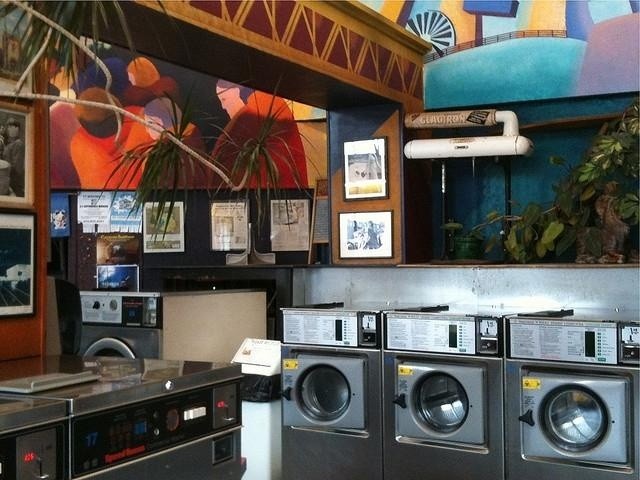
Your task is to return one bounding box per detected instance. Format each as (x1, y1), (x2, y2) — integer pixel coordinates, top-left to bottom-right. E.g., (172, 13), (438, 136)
(0, 0), (36, 101)
(0, 209), (38, 319)
(338, 209), (394, 259)
(141, 200), (187, 256)
(0, 99), (36, 210)
(341, 135), (389, 202)
(208, 199), (251, 254)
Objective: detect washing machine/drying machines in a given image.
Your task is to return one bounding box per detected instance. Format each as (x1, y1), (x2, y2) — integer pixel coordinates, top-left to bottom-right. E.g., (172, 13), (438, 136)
(77, 290), (162, 358)
(382, 306), (574, 480)
(504, 311), (640, 479)
(280, 303), (450, 480)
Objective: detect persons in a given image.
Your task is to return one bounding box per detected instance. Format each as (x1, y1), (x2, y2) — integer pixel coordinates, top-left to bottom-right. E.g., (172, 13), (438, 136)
(0, 118), (24, 197)
(349, 221), (382, 249)
